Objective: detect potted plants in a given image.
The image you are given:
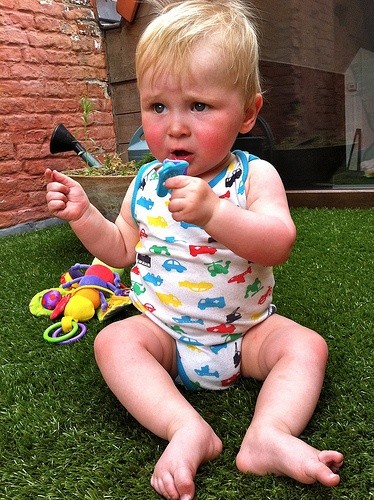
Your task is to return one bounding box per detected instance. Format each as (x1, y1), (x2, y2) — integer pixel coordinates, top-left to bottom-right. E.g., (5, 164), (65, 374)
(59, 96), (148, 223)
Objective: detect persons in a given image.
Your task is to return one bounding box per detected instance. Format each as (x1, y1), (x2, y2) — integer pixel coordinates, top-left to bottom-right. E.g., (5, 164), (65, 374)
(45, 0), (344, 500)
